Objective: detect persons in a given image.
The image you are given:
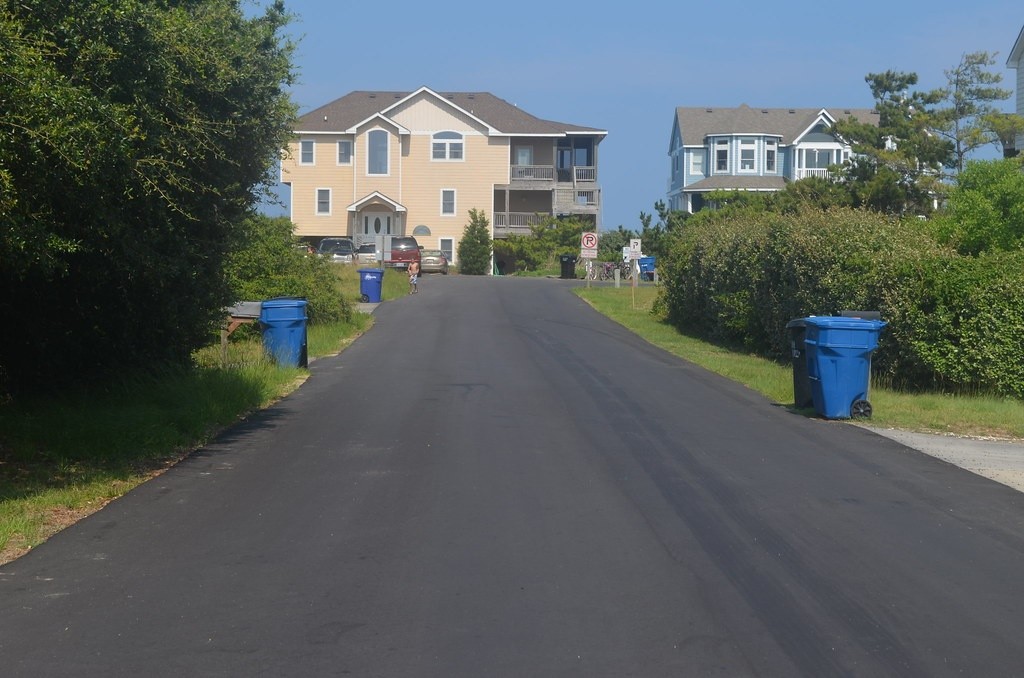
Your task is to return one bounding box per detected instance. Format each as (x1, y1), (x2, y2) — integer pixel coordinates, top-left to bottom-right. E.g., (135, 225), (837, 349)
(408, 256), (419, 295)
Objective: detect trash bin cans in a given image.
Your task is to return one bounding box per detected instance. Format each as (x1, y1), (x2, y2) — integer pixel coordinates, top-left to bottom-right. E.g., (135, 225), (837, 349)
(260, 297), (309, 369)
(806, 316), (889, 419)
(356, 268), (385, 303)
(638, 257), (656, 281)
(560, 256), (577, 279)
(786, 318), (814, 409)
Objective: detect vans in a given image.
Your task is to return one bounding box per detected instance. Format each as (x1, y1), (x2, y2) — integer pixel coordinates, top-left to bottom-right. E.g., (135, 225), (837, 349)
(317, 237), (359, 266)
(381, 234), (424, 277)
(354, 242), (381, 266)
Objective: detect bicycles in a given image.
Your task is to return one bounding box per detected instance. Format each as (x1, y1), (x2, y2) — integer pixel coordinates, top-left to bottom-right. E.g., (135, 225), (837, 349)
(599, 261), (633, 282)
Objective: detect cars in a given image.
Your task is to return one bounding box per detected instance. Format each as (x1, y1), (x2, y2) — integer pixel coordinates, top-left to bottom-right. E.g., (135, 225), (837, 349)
(419, 249), (448, 275)
